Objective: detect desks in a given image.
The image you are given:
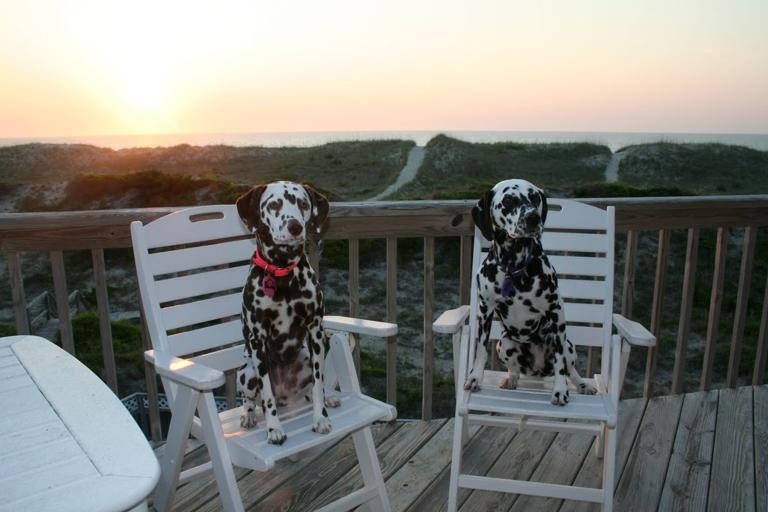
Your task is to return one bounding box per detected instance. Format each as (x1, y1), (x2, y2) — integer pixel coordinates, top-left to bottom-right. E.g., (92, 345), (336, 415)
(1, 333), (163, 512)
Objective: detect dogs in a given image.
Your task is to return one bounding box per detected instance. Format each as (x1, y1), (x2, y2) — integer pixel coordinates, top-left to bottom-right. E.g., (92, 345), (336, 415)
(236, 181), (341, 445)
(464, 179), (597, 406)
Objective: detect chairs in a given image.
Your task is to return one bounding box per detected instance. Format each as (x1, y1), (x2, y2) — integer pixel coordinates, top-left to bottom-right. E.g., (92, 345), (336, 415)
(431, 199), (656, 512)
(130, 202), (399, 511)
